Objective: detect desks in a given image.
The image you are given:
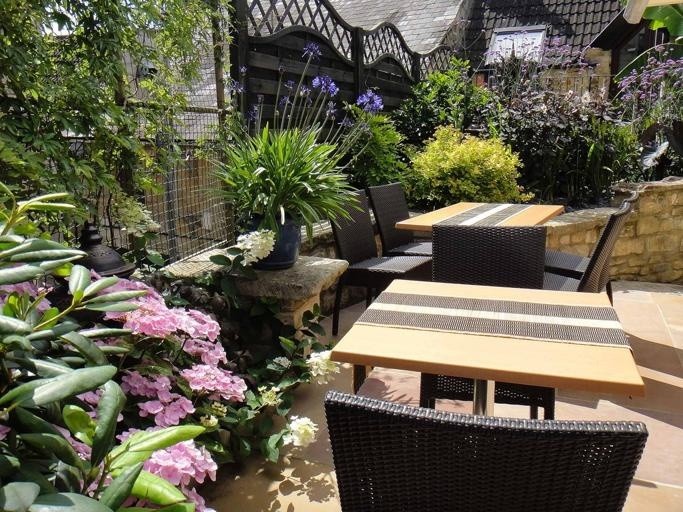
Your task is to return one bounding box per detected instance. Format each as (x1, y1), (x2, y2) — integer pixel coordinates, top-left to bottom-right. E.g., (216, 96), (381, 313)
(395, 200), (564, 234)
(329, 275), (647, 419)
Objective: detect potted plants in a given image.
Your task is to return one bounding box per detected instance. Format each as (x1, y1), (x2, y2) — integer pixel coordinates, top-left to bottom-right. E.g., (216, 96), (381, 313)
(197, 122), (368, 270)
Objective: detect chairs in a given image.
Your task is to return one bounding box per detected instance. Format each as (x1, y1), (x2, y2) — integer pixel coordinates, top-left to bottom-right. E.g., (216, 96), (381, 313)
(366, 180), (434, 259)
(543, 201), (632, 295)
(323, 186), (432, 334)
(416, 223), (557, 420)
(323, 387), (648, 511)
(542, 188), (638, 309)
